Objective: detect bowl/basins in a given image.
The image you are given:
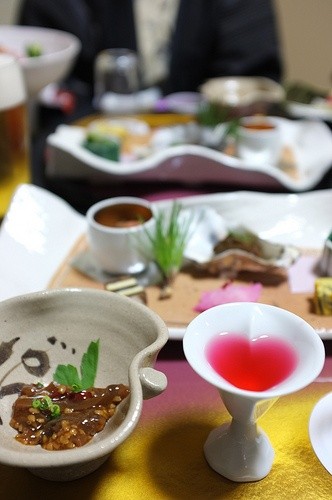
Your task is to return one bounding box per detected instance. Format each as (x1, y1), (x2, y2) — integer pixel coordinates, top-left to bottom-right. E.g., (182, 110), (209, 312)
(0, 26), (81, 94)
(202, 77), (285, 121)
(0, 288), (168, 481)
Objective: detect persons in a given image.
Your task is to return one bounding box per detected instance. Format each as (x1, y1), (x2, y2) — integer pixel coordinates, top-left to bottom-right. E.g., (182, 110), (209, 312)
(13, 0), (286, 181)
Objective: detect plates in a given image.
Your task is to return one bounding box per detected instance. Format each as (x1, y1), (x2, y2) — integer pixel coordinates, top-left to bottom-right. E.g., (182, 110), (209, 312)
(309, 392), (332, 476)
(47, 115), (332, 192)
(162, 206), (301, 273)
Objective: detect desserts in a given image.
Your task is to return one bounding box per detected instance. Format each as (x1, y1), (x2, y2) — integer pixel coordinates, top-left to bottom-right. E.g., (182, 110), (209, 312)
(313, 277), (331, 314)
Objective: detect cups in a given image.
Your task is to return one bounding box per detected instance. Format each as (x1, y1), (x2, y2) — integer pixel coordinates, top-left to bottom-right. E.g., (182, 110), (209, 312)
(86, 196), (160, 274)
(94, 49), (138, 111)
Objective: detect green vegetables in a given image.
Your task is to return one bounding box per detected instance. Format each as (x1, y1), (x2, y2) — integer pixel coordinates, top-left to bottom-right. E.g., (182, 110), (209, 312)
(32, 340), (98, 416)
(83, 104), (240, 164)
(140, 203), (206, 282)
(26, 47), (42, 58)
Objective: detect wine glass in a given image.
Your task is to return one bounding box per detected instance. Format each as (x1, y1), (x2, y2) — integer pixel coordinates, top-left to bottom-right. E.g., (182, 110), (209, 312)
(183, 301), (325, 482)
